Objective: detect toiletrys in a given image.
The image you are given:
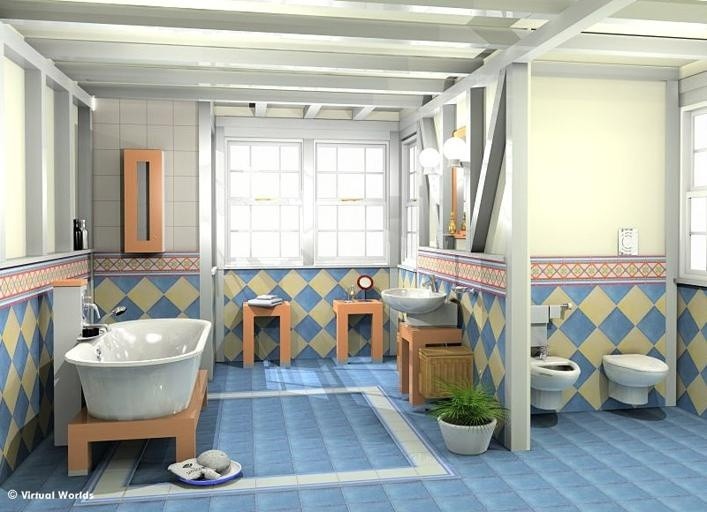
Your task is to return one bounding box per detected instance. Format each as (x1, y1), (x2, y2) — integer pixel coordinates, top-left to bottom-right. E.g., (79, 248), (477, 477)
(80, 219), (89, 249)
(73, 219), (83, 250)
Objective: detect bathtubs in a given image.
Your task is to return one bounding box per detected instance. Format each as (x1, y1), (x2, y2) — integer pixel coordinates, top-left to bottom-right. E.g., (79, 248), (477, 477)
(63, 318), (212, 421)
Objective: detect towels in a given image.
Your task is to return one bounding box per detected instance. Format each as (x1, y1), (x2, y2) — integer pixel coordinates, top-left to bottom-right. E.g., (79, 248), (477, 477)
(530, 305), (548, 347)
(248, 294), (282, 307)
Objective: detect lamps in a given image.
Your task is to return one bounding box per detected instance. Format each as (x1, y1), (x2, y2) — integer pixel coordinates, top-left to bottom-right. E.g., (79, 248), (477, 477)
(419, 148), (442, 176)
(443, 137), (466, 168)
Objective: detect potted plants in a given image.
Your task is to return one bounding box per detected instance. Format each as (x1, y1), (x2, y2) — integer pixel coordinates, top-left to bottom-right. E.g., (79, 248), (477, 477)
(427, 376), (511, 455)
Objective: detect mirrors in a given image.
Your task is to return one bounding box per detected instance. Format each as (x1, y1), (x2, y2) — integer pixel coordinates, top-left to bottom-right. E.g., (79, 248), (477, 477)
(357, 274), (374, 302)
(451, 127), (467, 236)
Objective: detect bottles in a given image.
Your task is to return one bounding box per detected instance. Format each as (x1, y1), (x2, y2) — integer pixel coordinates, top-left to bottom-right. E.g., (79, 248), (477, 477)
(74, 219), (89, 250)
(461, 212), (466, 231)
(348, 285), (355, 301)
(449, 212), (456, 234)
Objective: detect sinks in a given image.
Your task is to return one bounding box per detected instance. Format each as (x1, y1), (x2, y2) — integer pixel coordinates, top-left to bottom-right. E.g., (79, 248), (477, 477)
(382, 288), (446, 315)
(531, 357), (581, 411)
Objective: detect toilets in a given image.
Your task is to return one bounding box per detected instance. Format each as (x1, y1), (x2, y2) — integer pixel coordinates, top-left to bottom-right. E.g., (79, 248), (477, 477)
(603, 353), (668, 406)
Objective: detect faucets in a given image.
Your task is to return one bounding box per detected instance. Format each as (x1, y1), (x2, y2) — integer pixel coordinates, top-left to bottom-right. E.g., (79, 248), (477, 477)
(536, 345), (551, 361)
(84, 303), (101, 319)
(423, 275), (438, 292)
